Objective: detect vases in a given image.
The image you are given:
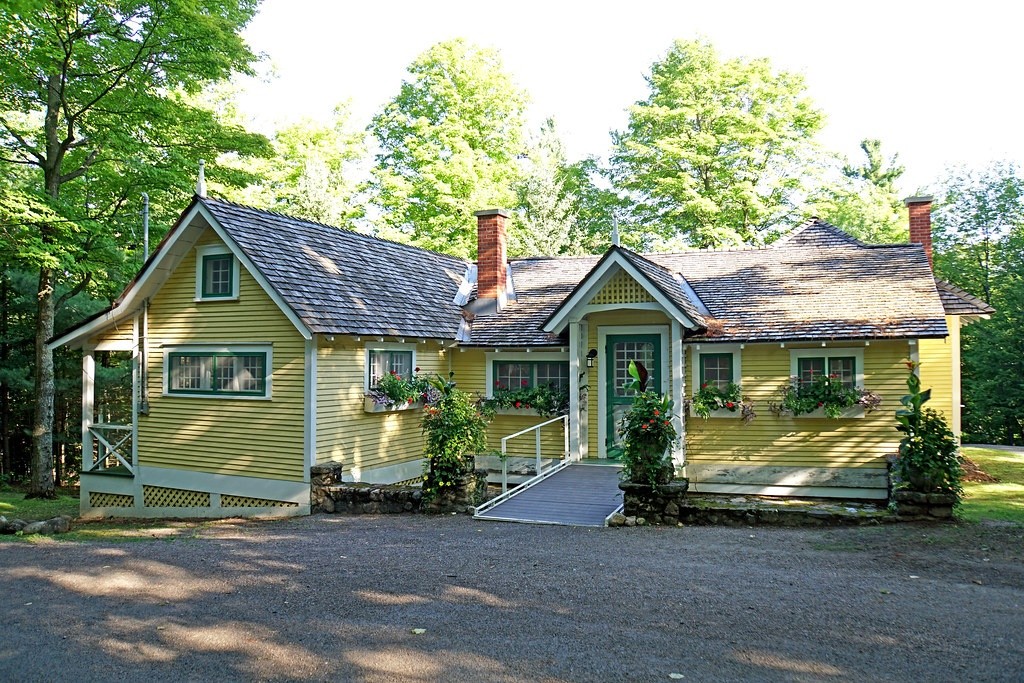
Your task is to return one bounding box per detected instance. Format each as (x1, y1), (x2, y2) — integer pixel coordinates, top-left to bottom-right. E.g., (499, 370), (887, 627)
(443, 438), (468, 455)
(636, 440), (668, 462)
(908, 472), (937, 491)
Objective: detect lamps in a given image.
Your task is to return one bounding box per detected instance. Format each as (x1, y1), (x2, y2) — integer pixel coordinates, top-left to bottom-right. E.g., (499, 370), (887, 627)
(585, 348), (597, 367)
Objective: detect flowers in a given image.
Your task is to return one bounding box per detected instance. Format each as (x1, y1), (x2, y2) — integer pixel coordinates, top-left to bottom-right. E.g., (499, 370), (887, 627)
(493, 378), (569, 432)
(367, 367), (434, 409)
(419, 385), (487, 512)
(888, 357), (967, 511)
(690, 380), (756, 426)
(617, 390), (677, 492)
(766, 369), (880, 419)
